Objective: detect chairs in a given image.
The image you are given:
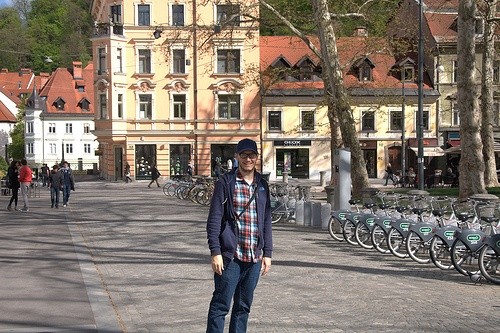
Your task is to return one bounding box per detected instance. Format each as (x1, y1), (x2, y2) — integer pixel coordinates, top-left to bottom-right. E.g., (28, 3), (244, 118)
(442, 176), (455, 188)
(406, 176), (414, 188)
(429, 176), (442, 189)
(1, 180), (12, 196)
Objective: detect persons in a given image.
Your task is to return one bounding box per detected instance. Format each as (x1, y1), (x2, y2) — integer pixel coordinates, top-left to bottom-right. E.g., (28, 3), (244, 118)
(186, 162), (192, 178)
(206, 138), (273, 333)
(7, 160), (23, 211)
(147, 165), (162, 188)
(18, 159), (32, 212)
(40, 161), (75, 209)
(408, 168), (416, 188)
(383, 163), (396, 186)
(445, 167), (456, 187)
(123, 162), (132, 183)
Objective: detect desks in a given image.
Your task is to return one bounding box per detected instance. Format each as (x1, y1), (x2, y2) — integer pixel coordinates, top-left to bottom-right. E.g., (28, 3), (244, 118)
(26, 181), (41, 199)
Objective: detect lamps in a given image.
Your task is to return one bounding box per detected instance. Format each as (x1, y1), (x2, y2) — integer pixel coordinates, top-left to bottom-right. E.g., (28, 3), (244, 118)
(44, 57), (54, 63)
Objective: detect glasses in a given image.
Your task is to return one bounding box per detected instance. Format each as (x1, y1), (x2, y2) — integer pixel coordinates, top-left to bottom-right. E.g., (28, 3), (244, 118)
(239, 153), (256, 159)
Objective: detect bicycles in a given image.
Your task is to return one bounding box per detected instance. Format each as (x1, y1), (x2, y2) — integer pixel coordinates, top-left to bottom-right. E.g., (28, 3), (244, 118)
(268, 183), (303, 224)
(328, 191), (500, 285)
(163, 175), (219, 207)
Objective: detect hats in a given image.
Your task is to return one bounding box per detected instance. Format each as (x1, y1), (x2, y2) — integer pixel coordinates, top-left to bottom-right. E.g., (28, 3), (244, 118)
(52, 165), (59, 170)
(236, 138), (258, 154)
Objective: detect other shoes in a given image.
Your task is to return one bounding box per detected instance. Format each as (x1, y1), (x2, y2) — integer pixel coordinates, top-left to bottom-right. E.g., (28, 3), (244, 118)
(63, 204), (67, 208)
(15, 206), (21, 211)
(66, 201), (70, 206)
(8, 206), (13, 212)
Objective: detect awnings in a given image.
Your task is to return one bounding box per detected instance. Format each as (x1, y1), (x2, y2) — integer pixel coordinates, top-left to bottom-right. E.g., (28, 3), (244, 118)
(409, 147), (445, 157)
